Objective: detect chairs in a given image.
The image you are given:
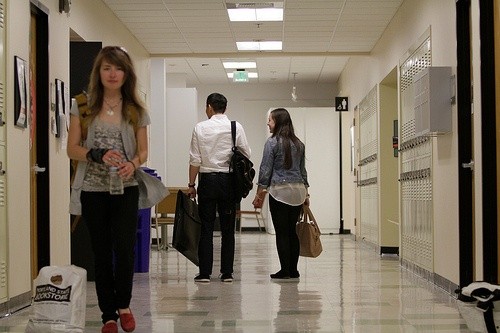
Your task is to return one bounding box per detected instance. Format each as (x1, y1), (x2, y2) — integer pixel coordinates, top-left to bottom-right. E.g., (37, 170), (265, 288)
(152, 186), (191, 251)
(234, 191), (269, 234)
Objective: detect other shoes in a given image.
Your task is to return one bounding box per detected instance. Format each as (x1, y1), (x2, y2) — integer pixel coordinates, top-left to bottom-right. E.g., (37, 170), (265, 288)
(118, 307), (135, 332)
(270, 269), (290, 279)
(102, 322), (118, 333)
(194, 275), (210, 281)
(290, 271), (300, 277)
(221, 274), (233, 281)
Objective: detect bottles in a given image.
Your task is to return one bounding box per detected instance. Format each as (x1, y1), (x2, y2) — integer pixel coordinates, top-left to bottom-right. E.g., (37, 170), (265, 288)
(109, 144), (124, 195)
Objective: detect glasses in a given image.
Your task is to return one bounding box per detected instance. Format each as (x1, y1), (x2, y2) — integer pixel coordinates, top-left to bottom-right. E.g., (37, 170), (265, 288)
(101, 46), (127, 54)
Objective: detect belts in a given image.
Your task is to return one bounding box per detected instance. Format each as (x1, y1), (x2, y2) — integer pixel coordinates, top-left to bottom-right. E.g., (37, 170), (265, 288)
(201, 172), (232, 176)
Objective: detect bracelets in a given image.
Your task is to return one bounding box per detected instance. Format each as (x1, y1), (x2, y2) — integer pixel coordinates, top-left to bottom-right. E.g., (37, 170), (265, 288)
(128, 161), (136, 171)
(188, 183), (195, 187)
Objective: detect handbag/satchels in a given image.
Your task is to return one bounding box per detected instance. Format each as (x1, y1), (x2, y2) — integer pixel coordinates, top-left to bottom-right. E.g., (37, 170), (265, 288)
(25, 265), (86, 333)
(172, 189), (199, 266)
(230, 147), (256, 198)
(296, 204), (325, 257)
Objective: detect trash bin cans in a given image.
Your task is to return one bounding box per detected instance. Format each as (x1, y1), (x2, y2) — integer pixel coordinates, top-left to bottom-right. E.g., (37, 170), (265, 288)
(134, 167), (161, 273)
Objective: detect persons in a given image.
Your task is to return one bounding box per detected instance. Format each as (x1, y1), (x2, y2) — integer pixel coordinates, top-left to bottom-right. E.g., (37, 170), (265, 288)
(188, 93), (251, 283)
(67, 46), (150, 333)
(252, 108), (310, 280)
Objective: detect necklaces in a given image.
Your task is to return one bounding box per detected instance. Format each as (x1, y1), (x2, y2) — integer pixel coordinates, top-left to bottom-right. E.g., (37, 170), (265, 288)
(103, 98), (122, 115)
